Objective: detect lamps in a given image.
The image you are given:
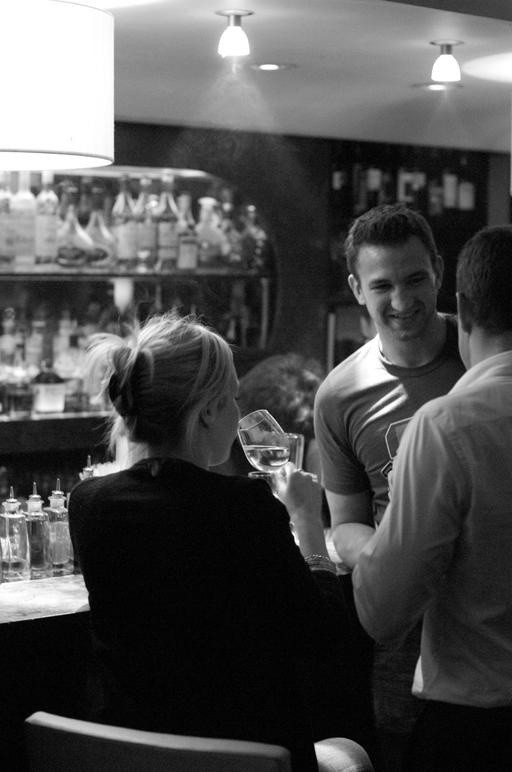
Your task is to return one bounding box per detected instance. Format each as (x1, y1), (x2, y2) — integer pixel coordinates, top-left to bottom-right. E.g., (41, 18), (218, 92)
(429, 40), (464, 82)
(215, 9), (255, 57)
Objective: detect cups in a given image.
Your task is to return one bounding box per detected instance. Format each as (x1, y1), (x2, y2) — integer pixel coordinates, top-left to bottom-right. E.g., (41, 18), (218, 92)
(285, 432), (304, 471)
(234, 407), (291, 477)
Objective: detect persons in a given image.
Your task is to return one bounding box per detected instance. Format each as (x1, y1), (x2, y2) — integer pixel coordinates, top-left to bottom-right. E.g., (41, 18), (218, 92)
(350, 223), (512, 772)
(64, 312), (346, 771)
(227, 340), (332, 528)
(311, 200), (469, 771)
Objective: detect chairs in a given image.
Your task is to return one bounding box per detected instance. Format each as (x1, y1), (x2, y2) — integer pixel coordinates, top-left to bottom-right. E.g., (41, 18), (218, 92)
(24, 711), (291, 772)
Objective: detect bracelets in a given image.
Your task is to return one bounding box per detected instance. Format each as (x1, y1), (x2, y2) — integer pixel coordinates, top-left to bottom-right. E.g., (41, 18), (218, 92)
(303, 549), (337, 576)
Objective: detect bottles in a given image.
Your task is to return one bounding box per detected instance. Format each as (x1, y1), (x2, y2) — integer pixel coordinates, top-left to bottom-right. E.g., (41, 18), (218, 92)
(31, 357), (67, 416)
(0, 167), (269, 276)
(0, 480), (75, 579)
(0, 302), (79, 365)
(4, 368), (34, 421)
(330, 152), (477, 218)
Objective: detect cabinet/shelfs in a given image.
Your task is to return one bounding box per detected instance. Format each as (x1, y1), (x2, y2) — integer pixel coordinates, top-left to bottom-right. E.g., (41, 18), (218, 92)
(0, 263), (271, 456)
